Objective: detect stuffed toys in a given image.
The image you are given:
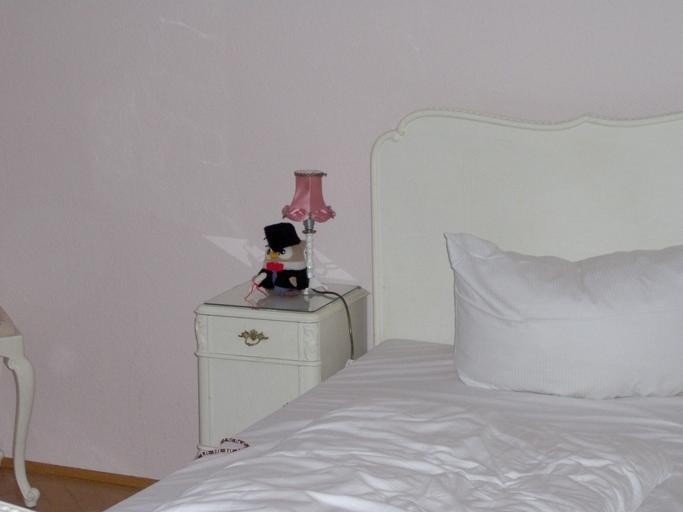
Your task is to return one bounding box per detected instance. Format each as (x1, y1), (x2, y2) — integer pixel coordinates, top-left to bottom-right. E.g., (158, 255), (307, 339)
(252, 221), (310, 296)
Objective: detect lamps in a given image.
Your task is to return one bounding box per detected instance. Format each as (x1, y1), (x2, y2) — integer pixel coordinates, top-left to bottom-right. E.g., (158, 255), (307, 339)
(282, 171), (336, 295)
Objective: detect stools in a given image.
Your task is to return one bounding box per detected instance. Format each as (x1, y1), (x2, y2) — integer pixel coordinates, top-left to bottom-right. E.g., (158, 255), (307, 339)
(1, 306), (39, 508)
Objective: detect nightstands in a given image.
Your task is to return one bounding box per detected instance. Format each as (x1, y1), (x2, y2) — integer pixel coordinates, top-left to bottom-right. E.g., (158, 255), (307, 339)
(194, 280), (370, 451)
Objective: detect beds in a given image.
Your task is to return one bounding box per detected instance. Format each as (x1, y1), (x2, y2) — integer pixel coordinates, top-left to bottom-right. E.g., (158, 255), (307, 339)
(102, 110), (683, 512)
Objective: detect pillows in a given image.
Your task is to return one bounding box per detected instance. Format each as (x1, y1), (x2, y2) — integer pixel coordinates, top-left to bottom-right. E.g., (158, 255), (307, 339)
(443, 232), (683, 399)
